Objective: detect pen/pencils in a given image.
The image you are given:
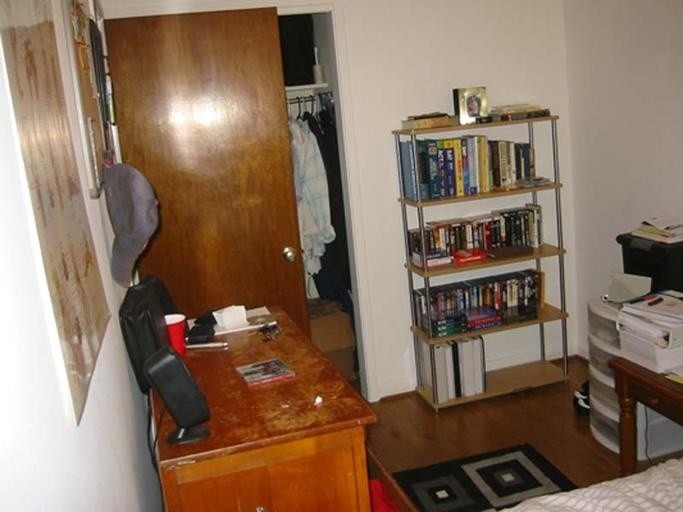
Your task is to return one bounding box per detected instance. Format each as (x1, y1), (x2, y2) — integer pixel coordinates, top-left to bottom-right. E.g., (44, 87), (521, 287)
(183, 340), (228, 350)
(629, 295), (656, 304)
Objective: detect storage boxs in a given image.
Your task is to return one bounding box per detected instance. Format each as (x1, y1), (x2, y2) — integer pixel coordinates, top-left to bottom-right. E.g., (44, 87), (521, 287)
(308, 300), (354, 381)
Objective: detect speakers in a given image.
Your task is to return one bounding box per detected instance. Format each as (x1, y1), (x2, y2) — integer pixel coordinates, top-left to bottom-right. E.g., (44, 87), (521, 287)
(139, 275), (190, 337)
(143, 344), (211, 444)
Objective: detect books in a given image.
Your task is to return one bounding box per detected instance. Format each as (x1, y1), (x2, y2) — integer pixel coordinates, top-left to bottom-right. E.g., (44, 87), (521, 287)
(400, 134), (536, 200)
(413, 268), (543, 338)
(630, 215), (683, 244)
(408, 203), (543, 268)
(402, 86), (549, 128)
(235, 359), (296, 388)
(420, 335), (487, 402)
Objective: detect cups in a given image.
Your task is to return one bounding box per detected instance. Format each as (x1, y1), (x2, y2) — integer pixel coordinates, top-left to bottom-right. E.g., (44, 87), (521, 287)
(157, 314), (186, 358)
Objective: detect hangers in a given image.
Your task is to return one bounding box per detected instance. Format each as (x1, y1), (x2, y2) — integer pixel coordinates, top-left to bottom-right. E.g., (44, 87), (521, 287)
(286, 93), (334, 145)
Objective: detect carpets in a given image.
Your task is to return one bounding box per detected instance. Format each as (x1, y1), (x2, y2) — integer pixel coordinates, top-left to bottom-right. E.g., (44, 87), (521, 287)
(391, 441), (579, 511)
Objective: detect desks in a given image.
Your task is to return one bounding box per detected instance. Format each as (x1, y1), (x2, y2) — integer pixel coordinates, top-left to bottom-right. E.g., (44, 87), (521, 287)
(148, 302), (378, 511)
(605, 356), (683, 477)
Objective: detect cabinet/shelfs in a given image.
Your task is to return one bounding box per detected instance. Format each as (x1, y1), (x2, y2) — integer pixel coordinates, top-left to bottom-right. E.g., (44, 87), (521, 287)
(391, 115), (571, 415)
(585, 296), (683, 464)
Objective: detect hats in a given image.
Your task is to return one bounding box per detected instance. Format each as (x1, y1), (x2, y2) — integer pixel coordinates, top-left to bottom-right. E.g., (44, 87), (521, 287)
(105, 164), (159, 287)
